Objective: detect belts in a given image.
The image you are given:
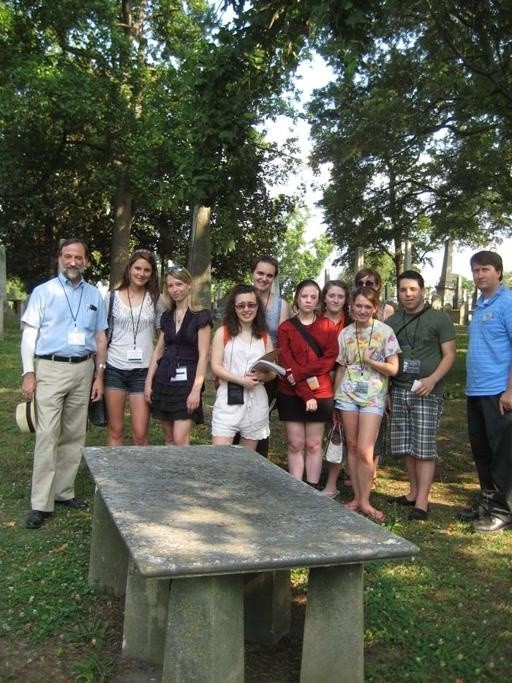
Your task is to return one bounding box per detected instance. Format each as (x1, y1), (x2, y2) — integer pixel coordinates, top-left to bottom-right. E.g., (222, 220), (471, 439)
(34, 354), (92, 364)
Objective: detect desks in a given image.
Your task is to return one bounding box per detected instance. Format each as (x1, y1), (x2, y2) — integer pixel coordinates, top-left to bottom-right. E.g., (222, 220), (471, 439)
(80, 444), (418, 683)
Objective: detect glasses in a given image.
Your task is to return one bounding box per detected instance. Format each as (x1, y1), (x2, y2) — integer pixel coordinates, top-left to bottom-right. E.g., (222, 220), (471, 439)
(165, 266), (191, 280)
(355, 280), (377, 289)
(234, 303), (259, 310)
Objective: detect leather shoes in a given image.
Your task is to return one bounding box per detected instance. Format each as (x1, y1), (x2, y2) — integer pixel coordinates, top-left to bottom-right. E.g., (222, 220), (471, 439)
(388, 494), (416, 506)
(458, 503), (485, 524)
(26, 510), (53, 529)
(54, 497), (88, 510)
(408, 503), (432, 521)
(472, 514), (512, 535)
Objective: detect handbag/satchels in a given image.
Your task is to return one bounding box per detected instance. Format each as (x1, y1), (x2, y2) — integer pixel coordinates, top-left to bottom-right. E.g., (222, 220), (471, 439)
(88, 395), (108, 428)
(226, 379), (245, 405)
(324, 422), (344, 464)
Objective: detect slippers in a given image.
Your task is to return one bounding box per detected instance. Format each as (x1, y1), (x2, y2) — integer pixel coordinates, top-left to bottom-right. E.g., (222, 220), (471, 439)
(342, 502), (361, 512)
(362, 503), (386, 523)
(316, 488), (340, 500)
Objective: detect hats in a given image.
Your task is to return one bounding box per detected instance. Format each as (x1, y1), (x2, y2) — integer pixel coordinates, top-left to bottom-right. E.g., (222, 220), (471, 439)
(15, 392), (36, 433)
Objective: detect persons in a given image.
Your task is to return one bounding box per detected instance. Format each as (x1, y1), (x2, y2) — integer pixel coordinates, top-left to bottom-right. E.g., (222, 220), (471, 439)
(458, 251), (512, 532)
(20, 239), (457, 529)
